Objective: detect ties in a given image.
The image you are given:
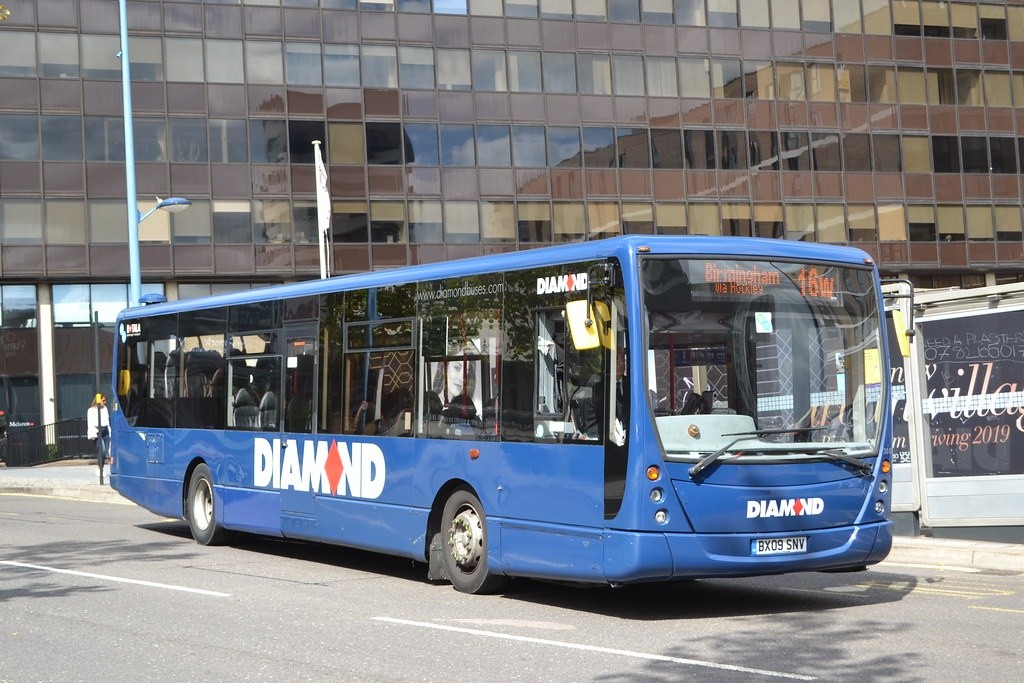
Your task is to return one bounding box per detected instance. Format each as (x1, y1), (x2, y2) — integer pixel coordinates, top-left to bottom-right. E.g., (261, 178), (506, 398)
(616, 381), (622, 397)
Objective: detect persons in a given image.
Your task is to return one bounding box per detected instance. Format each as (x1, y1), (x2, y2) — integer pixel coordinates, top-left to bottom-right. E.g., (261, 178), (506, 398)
(87, 394), (111, 478)
(584, 349), (628, 439)
(433, 347), (476, 407)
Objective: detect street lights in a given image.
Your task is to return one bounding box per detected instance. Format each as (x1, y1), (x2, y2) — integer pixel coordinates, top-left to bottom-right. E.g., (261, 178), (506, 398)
(129, 197), (192, 310)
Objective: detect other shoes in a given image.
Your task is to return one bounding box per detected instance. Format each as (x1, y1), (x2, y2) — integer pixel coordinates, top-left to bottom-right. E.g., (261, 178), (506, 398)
(95, 468), (106, 478)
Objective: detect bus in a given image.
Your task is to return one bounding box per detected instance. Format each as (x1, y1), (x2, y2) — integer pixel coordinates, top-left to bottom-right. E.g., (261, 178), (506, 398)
(109, 233), (916, 595)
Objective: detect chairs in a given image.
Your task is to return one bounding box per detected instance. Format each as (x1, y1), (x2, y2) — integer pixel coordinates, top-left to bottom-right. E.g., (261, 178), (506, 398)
(153, 347), (312, 433)
(388, 387), (498, 441)
